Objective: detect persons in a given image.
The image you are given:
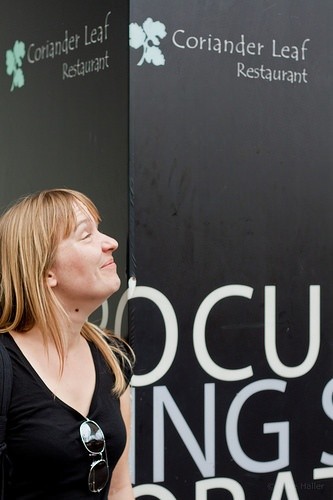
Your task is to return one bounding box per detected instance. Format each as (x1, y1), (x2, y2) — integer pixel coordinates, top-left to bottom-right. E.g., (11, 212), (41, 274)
(0, 186), (137, 500)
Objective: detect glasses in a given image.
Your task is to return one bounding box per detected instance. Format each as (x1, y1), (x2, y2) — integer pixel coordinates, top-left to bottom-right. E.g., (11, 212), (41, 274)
(79, 415), (111, 493)
